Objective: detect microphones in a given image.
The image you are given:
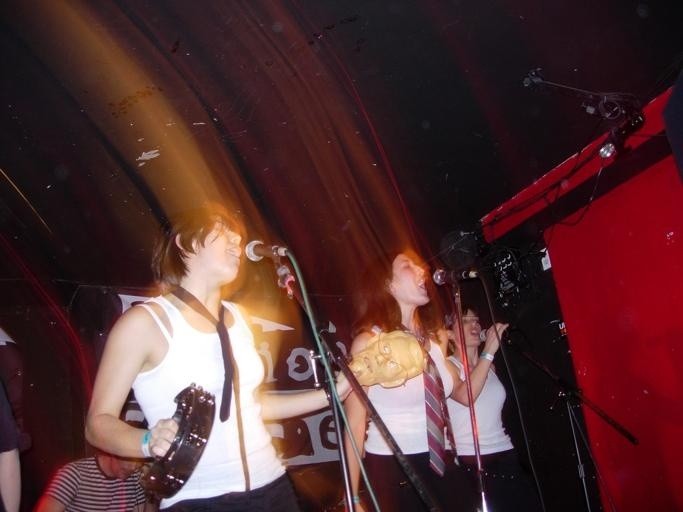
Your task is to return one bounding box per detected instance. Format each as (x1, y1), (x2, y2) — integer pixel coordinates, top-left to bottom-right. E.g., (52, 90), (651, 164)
(432, 268), (479, 287)
(478, 326), (518, 339)
(245, 239), (287, 264)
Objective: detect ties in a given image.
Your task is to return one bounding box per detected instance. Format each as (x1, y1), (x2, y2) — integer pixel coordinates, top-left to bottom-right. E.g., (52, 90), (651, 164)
(418, 333), (447, 476)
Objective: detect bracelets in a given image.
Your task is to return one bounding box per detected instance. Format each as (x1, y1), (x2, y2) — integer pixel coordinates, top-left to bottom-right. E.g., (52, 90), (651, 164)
(141, 430), (150, 458)
(336, 496), (361, 506)
(479, 352), (493, 362)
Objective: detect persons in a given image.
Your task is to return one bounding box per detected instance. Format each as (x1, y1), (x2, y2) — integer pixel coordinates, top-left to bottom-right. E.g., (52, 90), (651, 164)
(0, 379), (24, 511)
(33, 420), (158, 511)
(443, 307), (526, 512)
(339, 247), (453, 512)
(84, 202), (362, 512)
